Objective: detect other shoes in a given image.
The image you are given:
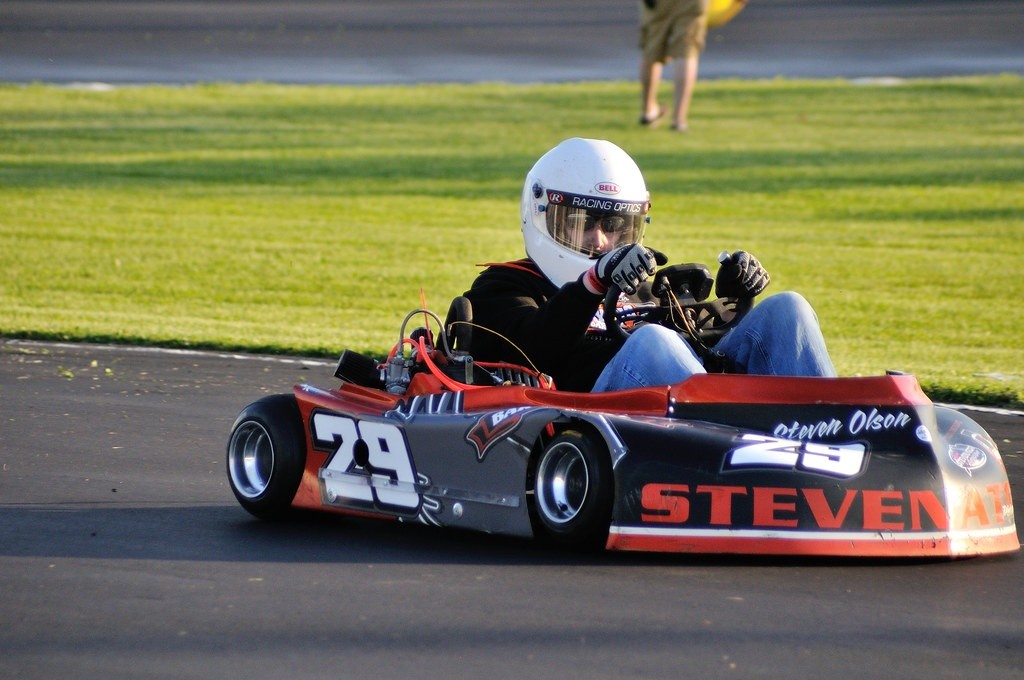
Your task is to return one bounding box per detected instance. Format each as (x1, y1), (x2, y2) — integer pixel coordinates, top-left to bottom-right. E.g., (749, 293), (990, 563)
(672, 123), (688, 132)
(640, 103), (664, 124)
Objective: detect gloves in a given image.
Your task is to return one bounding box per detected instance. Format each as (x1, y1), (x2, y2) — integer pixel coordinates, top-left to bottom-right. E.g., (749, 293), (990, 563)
(715, 250), (770, 308)
(595, 243), (668, 295)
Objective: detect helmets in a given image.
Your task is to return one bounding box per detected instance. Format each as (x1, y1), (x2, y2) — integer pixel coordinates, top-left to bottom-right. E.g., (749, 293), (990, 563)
(521, 138), (652, 291)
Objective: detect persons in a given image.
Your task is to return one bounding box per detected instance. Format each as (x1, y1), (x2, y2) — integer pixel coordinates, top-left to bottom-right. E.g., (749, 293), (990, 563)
(465, 138), (842, 395)
(636, 0), (711, 131)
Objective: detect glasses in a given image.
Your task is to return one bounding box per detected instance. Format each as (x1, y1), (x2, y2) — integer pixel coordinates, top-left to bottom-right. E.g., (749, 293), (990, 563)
(565, 212), (627, 233)
